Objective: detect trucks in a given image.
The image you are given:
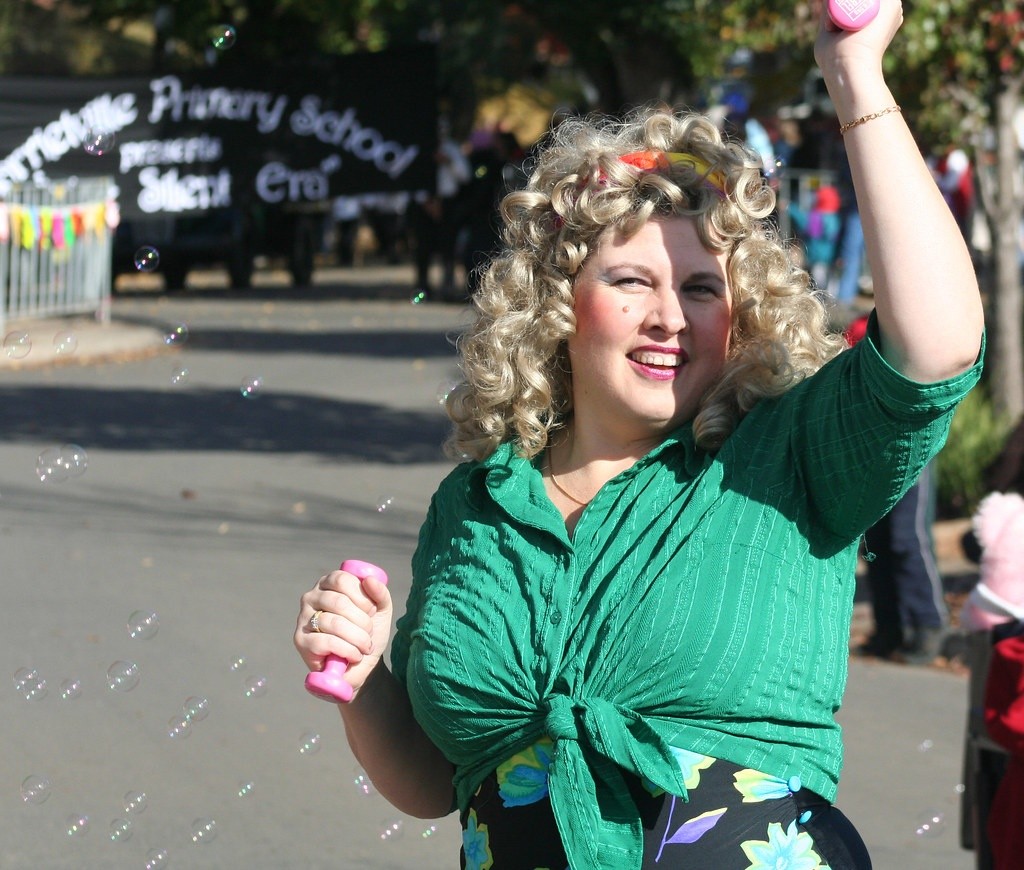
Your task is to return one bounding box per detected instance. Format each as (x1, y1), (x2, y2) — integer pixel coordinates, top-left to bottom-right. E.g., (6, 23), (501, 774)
(1, 63), (334, 292)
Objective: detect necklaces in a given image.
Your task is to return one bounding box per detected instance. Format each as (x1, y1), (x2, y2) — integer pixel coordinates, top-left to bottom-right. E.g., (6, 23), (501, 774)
(547, 438), (591, 506)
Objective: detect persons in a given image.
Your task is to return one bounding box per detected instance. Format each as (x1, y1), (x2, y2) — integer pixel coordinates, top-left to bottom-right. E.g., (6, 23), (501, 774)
(402, 132), (529, 300)
(292, 0), (986, 870)
(842, 271), (954, 664)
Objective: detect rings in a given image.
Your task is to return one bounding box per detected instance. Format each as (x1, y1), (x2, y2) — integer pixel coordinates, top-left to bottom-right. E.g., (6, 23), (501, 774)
(311, 611), (322, 632)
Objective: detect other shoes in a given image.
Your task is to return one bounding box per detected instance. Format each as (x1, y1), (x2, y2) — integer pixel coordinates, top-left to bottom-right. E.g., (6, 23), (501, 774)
(852, 623), (904, 657)
(890, 619), (949, 657)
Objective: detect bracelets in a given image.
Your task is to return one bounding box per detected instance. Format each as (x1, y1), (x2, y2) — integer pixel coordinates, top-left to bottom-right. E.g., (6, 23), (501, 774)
(839, 106), (902, 133)
